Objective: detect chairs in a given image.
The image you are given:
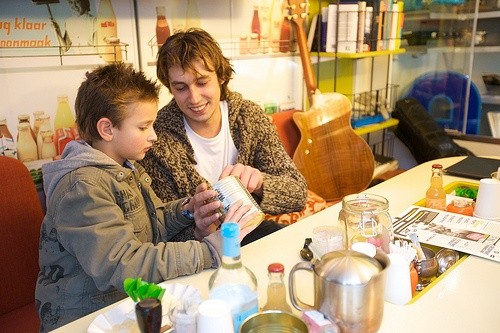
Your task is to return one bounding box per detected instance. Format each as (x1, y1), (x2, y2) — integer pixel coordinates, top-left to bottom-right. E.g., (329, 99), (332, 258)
(0, 157), (46, 333)
(264, 110), (304, 160)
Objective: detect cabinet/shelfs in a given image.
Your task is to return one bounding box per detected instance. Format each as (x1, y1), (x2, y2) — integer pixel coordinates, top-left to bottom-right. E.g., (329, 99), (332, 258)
(306, 47), (407, 179)
(399, 8), (500, 103)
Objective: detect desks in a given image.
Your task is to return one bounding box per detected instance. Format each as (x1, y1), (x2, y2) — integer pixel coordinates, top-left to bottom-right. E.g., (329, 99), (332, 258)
(47, 157), (500, 333)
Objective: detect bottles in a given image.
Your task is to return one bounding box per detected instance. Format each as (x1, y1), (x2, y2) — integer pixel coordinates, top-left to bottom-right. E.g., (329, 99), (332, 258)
(409, 263), (419, 298)
(96, 0), (123, 63)
(0, 94), (82, 165)
(425, 165), (446, 211)
(207, 223), (258, 333)
(239, 6), (298, 54)
(156, 0), (200, 53)
(136, 298), (162, 333)
(339, 193), (394, 254)
(263, 263), (292, 315)
(447, 203), (473, 216)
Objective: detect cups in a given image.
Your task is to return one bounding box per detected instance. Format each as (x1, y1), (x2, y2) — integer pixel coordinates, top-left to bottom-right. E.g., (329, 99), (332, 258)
(169, 297), (235, 333)
(473, 178), (500, 222)
(311, 225), (344, 258)
(205, 175), (265, 233)
(240, 311), (309, 333)
(289, 248), (390, 333)
(413, 247), (438, 291)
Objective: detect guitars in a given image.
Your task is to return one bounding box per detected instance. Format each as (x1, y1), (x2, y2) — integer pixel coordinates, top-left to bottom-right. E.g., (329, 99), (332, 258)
(285, 0), (375, 202)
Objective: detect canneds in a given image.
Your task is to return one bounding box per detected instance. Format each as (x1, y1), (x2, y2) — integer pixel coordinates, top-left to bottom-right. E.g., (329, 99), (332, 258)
(207, 175), (266, 230)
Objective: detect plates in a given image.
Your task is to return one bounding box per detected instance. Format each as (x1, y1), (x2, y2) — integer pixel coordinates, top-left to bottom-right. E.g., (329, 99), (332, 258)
(87, 283), (201, 333)
(444, 181), (480, 200)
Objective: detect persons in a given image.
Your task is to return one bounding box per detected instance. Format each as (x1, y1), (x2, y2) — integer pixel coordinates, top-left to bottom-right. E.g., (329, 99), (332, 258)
(135, 27), (308, 247)
(49, 0), (98, 55)
(34, 59), (261, 333)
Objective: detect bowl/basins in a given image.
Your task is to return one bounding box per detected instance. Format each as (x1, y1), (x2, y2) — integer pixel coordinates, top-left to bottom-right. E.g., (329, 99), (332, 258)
(405, 29), (487, 46)
